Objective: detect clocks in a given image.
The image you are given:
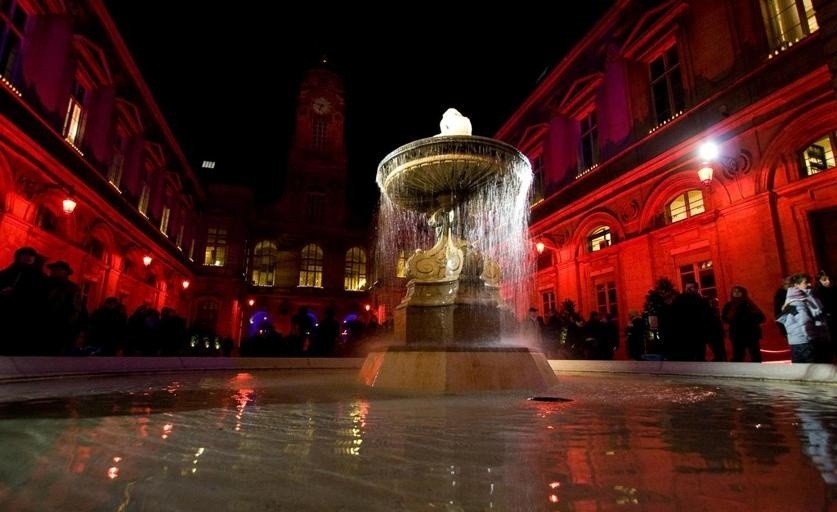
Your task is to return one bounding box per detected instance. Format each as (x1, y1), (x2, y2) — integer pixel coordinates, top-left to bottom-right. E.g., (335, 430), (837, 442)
(312, 97), (331, 114)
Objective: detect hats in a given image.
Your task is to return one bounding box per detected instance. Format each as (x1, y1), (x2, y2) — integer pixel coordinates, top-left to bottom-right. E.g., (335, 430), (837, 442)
(48, 261), (72, 275)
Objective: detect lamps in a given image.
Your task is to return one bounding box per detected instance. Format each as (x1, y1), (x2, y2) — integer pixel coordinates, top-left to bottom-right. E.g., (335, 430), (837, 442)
(698, 154), (738, 186)
(45, 182), (76, 216)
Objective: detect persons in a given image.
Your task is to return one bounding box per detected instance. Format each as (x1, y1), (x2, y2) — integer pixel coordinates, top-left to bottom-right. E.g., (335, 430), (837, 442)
(307, 308), (339, 357)
(0, 248), (231, 356)
(289, 306), (313, 356)
